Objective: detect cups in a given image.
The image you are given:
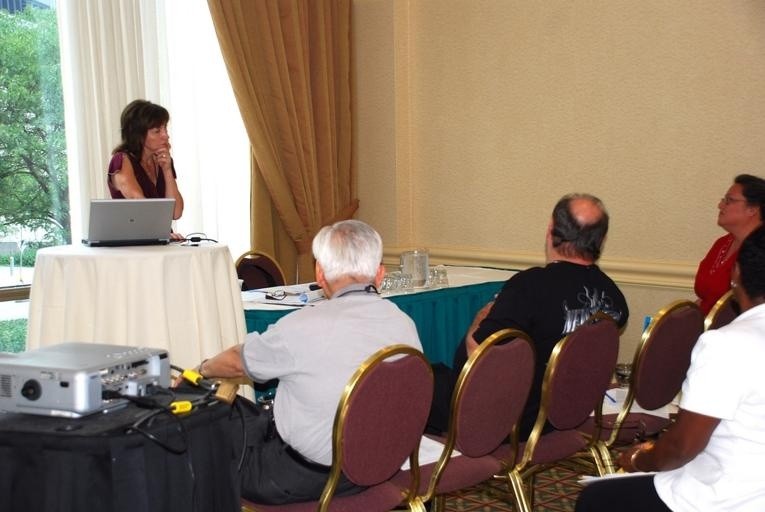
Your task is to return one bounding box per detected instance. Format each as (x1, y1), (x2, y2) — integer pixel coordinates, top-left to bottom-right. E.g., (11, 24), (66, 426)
(616, 363), (633, 387)
(379, 249), (449, 293)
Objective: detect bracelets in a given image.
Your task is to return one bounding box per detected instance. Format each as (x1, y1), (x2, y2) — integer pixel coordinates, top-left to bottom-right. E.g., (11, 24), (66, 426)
(198, 357), (209, 379)
(631, 445), (642, 475)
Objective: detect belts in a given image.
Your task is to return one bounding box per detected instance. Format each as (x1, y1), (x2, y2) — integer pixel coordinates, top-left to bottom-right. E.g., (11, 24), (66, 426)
(277, 433), (331, 474)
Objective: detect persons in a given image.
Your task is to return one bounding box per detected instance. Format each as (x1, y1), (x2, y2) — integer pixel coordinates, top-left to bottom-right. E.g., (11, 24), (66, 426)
(574, 223), (765, 511)
(106, 99), (187, 244)
(172, 220), (424, 506)
(414, 193), (631, 437)
(694, 174), (765, 317)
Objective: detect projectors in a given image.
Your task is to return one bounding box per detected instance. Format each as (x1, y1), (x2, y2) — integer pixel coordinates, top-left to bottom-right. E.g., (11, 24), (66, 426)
(0, 342), (170, 419)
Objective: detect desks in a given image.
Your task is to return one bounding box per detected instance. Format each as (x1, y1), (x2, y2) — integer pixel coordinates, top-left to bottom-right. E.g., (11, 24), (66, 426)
(20, 243), (257, 406)
(239, 262), (526, 402)
(0, 384), (249, 511)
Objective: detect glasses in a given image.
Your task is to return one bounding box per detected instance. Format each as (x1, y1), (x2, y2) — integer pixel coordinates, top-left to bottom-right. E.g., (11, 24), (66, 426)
(265, 290), (300, 300)
(721, 197), (745, 205)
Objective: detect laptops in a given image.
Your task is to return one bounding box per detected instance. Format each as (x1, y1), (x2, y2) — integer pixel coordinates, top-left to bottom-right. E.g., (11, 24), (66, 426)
(81, 198), (176, 247)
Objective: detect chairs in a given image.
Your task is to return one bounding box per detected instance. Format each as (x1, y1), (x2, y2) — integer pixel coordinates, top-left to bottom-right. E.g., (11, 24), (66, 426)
(427, 308), (619, 512)
(705, 288), (739, 330)
(240, 341), (434, 509)
(571, 300), (706, 479)
(389, 325), (535, 512)
(232, 248), (286, 290)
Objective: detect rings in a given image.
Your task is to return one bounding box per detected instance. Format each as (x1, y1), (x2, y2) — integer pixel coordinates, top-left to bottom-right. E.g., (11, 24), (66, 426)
(162, 154), (166, 159)
